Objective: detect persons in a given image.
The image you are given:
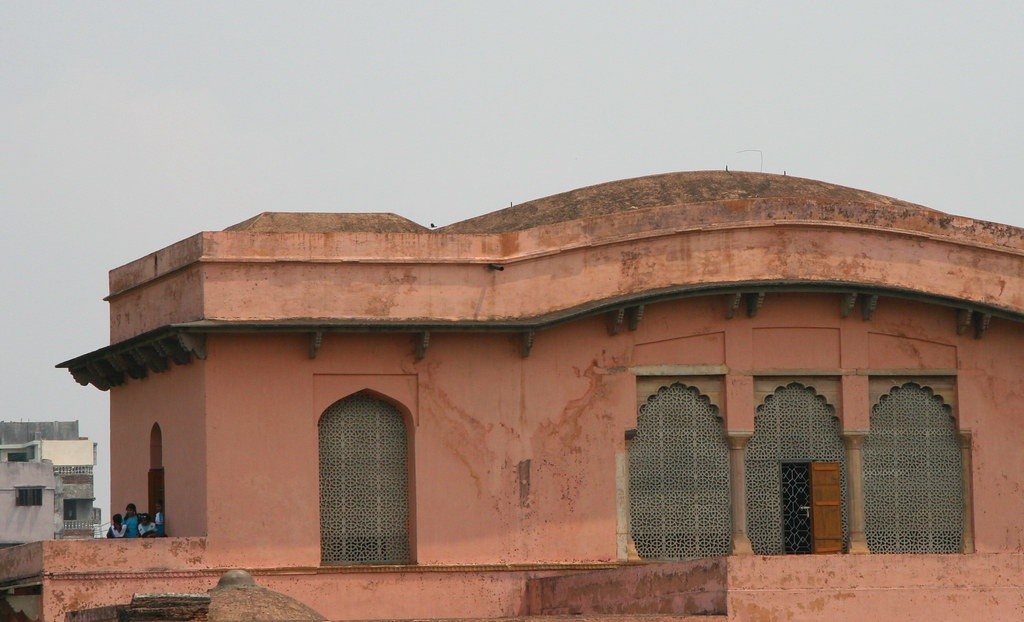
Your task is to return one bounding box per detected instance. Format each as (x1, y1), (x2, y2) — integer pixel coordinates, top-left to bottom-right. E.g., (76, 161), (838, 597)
(107, 514), (129, 538)
(122, 503), (140, 538)
(137, 513), (156, 536)
(154, 500), (164, 536)
